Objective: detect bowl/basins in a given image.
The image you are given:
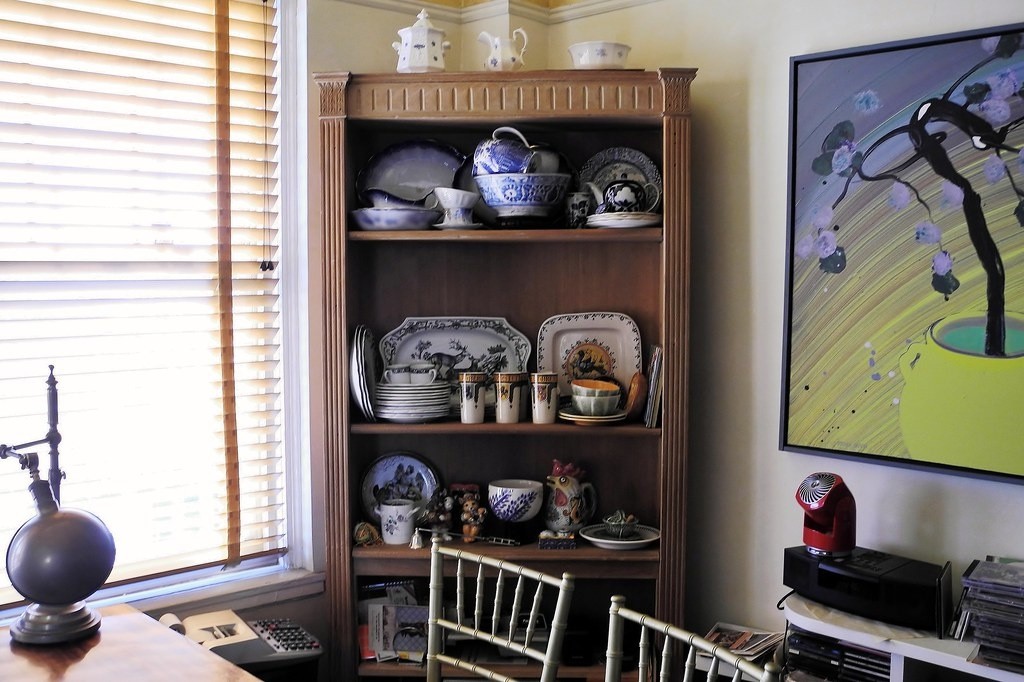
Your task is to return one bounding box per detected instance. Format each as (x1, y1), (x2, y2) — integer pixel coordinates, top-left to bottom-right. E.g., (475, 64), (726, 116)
(603, 516), (639, 537)
(567, 41), (632, 70)
(569, 379), (620, 396)
(474, 174), (571, 217)
(570, 394), (621, 416)
(487, 479), (543, 521)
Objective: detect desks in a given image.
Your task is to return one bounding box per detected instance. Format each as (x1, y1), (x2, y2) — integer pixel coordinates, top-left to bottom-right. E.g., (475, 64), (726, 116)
(0, 600), (268, 682)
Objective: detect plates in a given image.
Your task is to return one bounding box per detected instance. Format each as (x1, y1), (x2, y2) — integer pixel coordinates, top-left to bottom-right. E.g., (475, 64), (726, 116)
(350, 140), (664, 231)
(376, 380), (451, 424)
(537, 311), (646, 426)
(349, 323), (379, 422)
(358, 452), (660, 549)
(380, 317), (532, 416)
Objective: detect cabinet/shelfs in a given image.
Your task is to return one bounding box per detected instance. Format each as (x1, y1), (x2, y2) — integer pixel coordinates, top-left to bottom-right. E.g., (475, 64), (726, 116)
(783, 591), (1024, 682)
(313, 67), (702, 682)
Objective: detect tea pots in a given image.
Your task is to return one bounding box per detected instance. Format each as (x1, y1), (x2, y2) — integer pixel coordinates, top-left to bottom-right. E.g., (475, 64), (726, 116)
(584, 174), (659, 212)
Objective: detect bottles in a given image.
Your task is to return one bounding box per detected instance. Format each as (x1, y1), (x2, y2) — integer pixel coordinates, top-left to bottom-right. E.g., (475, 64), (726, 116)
(392, 9), (452, 74)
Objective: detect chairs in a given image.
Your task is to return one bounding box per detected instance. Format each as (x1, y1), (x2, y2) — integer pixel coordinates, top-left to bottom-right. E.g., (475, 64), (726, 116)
(425, 534), (781, 682)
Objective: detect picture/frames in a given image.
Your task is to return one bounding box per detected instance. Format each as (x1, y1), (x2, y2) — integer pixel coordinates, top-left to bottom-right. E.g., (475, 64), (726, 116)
(778, 21), (1023, 486)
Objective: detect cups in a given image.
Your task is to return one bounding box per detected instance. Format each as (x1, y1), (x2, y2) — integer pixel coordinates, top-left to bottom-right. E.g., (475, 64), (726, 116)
(531, 373), (558, 423)
(472, 126), (559, 175)
(375, 499), (423, 545)
(383, 359), (438, 384)
(493, 371), (529, 423)
(457, 371), (486, 424)
(566, 192), (591, 228)
(432, 187), (485, 229)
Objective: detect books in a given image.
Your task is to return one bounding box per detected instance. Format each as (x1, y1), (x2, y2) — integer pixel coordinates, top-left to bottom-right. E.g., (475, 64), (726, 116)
(696, 630), (784, 660)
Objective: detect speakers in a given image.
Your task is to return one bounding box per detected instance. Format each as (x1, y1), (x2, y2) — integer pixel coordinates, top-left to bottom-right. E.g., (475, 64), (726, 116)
(782, 546), (819, 601)
(879, 559), (941, 633)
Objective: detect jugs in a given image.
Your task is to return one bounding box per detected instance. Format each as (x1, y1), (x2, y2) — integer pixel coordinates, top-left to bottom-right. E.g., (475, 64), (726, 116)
(478, 27), (528, 72)
